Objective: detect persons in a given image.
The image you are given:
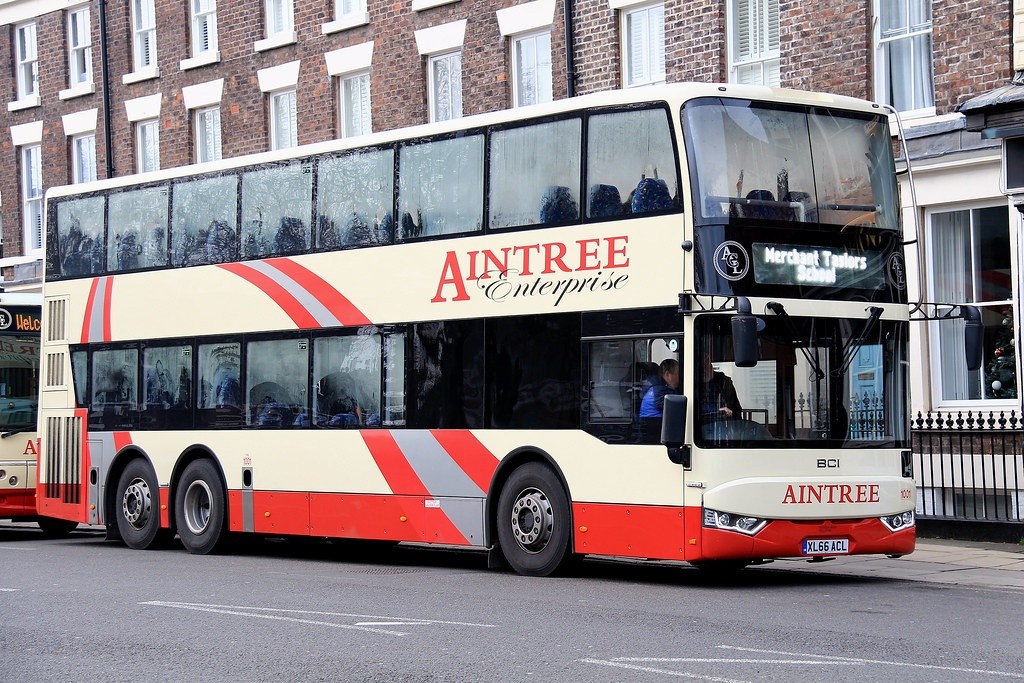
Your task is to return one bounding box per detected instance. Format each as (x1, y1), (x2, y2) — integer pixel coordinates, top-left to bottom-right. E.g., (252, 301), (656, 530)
(703, 354), (745, 419)
(640, 359), (733, 419)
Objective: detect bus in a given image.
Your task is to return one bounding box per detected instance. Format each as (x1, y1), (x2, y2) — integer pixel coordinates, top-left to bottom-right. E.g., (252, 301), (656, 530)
(0, 288), (81, 536)
(34, 79), (984, 577)
